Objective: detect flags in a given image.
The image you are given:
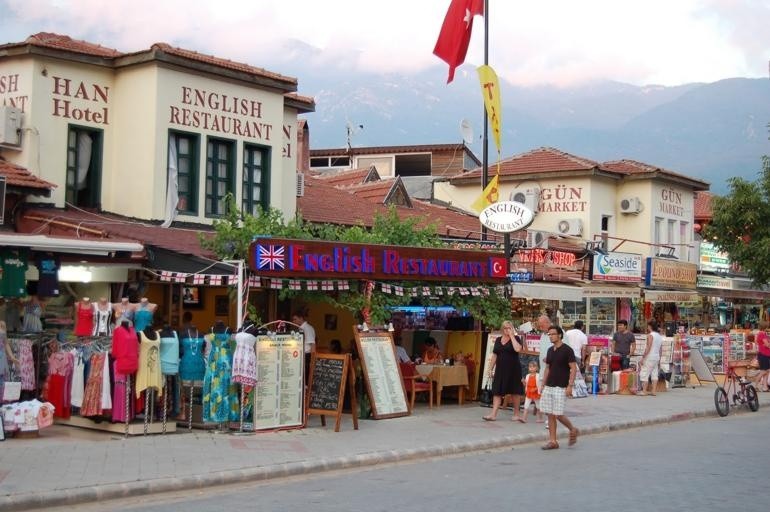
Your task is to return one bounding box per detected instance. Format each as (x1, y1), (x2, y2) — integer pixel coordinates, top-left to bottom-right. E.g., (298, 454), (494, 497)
(433, 0), (485, 84)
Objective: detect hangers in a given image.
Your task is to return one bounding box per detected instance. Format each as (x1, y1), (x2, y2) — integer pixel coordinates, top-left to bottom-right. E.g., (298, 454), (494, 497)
(56, 338), (108, 355)
(8, 332), (32, 343)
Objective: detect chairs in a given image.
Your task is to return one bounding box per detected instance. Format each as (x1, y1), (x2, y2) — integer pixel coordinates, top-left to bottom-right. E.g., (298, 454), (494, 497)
(399, 363), (434, 410)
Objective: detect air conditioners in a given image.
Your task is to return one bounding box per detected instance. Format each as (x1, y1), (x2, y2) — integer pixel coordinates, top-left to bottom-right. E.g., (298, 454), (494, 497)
(556, 219), (582, 237)
(296, 169), (304, 197)
(508, 188), (540, 212)
(0, 106), (22, 146)
(527, 230), (550, 249)
(619, 197), (640, 214)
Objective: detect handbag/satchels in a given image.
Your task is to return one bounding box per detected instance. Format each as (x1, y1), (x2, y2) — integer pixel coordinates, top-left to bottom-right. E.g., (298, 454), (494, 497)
(480, 389), (493, 403)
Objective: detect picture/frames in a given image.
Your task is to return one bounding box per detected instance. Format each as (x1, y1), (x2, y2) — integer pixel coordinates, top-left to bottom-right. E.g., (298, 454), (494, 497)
(214, 295), (229, 316)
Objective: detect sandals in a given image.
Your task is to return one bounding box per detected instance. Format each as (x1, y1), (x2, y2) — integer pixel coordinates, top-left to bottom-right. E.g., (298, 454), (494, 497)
(541, 442), (560, 450)
(568, 430), (579, 445)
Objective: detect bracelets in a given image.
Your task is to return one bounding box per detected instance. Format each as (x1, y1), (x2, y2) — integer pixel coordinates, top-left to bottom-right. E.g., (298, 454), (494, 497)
(569, 382), (575, 387)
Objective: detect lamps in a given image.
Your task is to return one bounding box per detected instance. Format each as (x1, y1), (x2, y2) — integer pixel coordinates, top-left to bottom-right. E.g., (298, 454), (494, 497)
(362, 321), (369, 331)
(388, 320), (395, 332)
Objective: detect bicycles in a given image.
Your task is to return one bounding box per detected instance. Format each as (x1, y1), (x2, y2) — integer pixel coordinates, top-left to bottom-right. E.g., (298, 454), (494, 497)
(714, 355), (759, 416)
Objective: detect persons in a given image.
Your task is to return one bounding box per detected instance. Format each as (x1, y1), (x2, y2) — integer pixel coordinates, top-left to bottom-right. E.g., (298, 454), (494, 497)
(612, 320), (636, 372)
(74, 295), (260, 375)
(684, 323), (688, 332)
(755, 320), (769, 392)
(330, 339), (347, 353)
(183, 288), (194, 301)
(538, 315), (570, 428)
(482, 319), (523, 421)
(636, 321), (662, 396)
(394, 335), (410, 362)
(517, 360), (543, 422)
(0, 324), (20, 380)
(534, 326), (579, 450)
(18, 294), (42, 335)
(422, 337), (442, 363)
(292, 311), (316, 388)
(179, 310), (202, 338)
(566, 320), (588, 396)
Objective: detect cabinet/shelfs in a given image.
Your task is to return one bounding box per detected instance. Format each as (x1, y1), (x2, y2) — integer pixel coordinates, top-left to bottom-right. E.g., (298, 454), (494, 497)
(586, 328), (747, 389)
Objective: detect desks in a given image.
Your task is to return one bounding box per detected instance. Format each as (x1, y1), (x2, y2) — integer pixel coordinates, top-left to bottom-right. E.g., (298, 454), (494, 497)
(415, 364), (469, 407)
